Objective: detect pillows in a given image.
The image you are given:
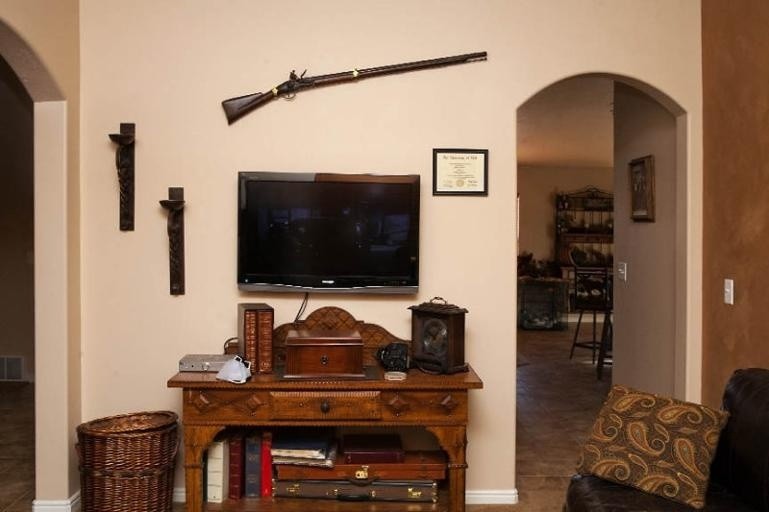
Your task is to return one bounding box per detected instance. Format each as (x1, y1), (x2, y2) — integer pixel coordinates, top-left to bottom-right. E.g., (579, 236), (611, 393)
(574, 384), (731, 509)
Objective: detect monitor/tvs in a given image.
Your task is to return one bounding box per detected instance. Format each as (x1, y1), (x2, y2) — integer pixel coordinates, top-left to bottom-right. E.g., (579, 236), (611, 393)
(236, 170), (421, 296)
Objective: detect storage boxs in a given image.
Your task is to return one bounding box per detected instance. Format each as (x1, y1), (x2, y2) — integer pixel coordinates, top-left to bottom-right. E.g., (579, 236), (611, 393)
(271, 450), (449, 503)
(284, 331), (366, 377)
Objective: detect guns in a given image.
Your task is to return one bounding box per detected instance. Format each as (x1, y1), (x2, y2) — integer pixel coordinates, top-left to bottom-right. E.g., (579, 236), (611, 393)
(221, 51), (486, 125)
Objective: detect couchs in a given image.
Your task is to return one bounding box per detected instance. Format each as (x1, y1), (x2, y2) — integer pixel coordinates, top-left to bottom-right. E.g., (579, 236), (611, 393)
(561, 368), (769, 511)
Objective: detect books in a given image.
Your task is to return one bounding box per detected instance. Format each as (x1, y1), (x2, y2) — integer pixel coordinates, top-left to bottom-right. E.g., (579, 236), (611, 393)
(206, 428), (338, 504)
(343, 433), (405, 463)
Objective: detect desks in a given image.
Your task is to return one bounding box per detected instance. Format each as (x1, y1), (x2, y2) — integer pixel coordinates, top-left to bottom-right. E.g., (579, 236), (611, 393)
(518, 275), (571, 330)
(167, 306), (483, 512)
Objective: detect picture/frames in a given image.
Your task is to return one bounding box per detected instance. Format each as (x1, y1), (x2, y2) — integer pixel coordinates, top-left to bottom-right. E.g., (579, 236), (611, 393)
(628, 155), (656, 223)
(432, 148), (488, 197)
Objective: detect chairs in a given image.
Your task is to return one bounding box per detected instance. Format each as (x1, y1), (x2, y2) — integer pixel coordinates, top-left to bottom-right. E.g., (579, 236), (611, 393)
(568, 265), (613, 379)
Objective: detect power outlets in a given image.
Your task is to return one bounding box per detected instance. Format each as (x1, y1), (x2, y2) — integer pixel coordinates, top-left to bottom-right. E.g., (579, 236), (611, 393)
(618, 261), (627, 281)
(723, 279), (734, 305)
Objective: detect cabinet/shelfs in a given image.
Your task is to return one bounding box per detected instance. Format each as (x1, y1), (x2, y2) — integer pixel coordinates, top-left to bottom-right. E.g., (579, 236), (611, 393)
(555, 185), (614, 312)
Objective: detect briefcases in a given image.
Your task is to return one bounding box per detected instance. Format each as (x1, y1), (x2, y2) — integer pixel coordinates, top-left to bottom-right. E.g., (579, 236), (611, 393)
(272, 451), (445, 503)
